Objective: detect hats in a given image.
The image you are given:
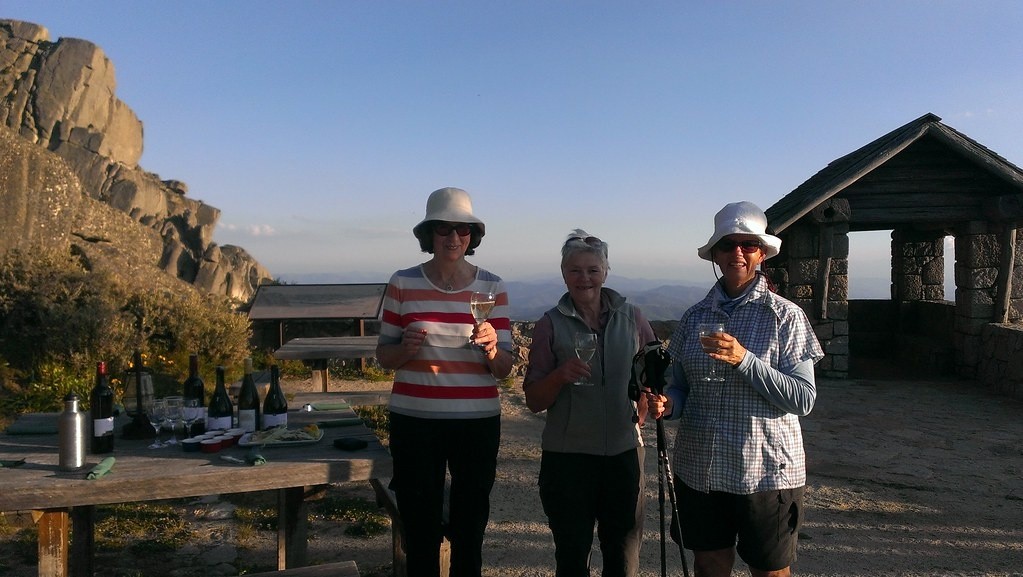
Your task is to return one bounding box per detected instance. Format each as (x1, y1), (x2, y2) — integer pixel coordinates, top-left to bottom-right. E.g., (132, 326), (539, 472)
(413, 187), (485, 248)
(698, 201), (782, 261)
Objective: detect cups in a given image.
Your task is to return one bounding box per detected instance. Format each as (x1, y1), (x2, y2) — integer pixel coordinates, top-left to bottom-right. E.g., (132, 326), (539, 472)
(303, 403), (312, 412)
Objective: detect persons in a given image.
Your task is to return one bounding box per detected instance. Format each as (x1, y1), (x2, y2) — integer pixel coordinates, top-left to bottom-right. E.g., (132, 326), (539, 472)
(374, 189), (513, 577)
(521, 231), (656, 577)
(645, 202), (824, 577)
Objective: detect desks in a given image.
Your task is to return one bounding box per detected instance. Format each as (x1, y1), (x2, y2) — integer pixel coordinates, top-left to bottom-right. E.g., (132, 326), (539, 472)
(0, 399), (393, 577)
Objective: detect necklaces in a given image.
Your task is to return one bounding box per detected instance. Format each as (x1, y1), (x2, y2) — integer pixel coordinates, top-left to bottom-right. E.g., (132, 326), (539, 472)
(447, 284), (453, 290)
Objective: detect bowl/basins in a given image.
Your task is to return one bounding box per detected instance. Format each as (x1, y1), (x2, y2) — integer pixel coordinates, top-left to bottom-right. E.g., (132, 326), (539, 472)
(179, 428), (247, 452)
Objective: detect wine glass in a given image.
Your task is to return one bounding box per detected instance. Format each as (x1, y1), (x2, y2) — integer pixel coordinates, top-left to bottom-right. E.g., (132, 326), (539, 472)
(163, 396), (185, 445)
(146, 398), (169, 450)
(470, 292), (496, 347)
(177, 398), (200, 443)
(699, 323), (726, 382)
(573, 333), (597, 387)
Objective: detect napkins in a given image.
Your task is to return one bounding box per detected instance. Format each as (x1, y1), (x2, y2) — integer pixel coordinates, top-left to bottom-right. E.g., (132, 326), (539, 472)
(318, 419), (362, 427)
(0, 460), (26, 467)
(249, 452), (266, 466)
(6, 428), (58, 434)
(86, 457), (116, 479)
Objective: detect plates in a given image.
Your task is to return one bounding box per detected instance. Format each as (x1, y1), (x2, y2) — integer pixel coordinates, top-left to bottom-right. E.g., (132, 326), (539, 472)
(238, 430), (324, 446)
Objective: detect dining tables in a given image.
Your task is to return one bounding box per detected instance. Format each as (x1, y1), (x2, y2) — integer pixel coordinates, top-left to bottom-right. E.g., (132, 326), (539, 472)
(314, 403), (349, 410)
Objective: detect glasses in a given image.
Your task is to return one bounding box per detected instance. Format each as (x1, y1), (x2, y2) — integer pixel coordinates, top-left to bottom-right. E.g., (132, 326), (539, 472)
(562, 237), (607, 259)
(714, 239), (762, 253)
(435, 223), (473, 237)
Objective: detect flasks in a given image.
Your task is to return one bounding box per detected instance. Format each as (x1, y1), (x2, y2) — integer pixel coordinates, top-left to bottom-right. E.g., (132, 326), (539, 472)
(57, 393), (88, 472)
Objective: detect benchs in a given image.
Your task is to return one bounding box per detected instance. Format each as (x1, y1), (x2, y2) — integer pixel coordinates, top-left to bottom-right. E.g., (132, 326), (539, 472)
(294, 391), (391, 404)
(229, 370), (283, 395)
(243, 560), (360, 577)
(369, 477), (452, 577)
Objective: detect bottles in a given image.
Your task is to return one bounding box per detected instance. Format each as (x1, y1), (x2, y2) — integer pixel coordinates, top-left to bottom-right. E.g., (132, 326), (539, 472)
(238, 358), (260, 434)
(263, 365), (288, 431)
(184, 355), (205, 439)
(207, 366), (233, 431)
(90, 361), (115, 454)
